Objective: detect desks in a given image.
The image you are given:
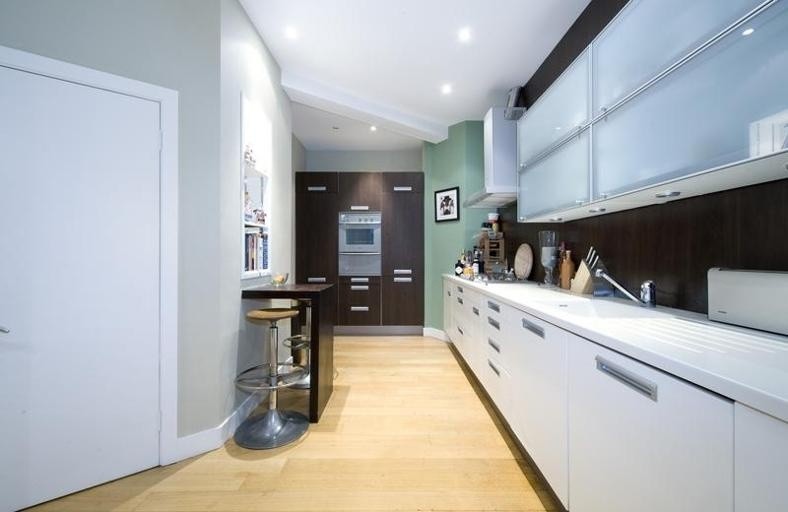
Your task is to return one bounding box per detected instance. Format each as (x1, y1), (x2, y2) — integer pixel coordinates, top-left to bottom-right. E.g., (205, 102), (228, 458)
(244, 277), (337, 422)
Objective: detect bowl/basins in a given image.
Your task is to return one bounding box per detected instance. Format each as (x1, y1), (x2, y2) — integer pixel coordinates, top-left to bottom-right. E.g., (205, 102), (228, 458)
(267, 271), (288, 286)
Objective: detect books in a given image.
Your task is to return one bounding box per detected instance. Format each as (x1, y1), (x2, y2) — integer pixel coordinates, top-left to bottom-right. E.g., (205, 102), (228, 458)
(245, 172), (270, 272)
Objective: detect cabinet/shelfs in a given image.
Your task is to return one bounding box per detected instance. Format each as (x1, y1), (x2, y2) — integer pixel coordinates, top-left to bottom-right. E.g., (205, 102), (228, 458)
(238, 158), (272, 281)
(513, 0), (787, 225)
(568, 331), (734, 511)
(339, 278), (383, 327)
(294, 171), (338, 276)
(383, 276), (424, 326)
(383, 173), (424, 275)
(501, 300), (568, 509)
(338, 173), (383, 213)
(440, 272), (502, 412)
(735, 399), (788, 511)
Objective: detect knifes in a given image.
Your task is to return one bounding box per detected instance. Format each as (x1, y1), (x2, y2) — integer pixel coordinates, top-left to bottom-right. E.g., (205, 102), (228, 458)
(584, 244), (599, 270)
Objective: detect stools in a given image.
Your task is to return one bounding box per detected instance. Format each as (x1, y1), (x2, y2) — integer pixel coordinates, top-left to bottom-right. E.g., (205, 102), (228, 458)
(283, 298), (313, 390)
(235, 306), (311, 449)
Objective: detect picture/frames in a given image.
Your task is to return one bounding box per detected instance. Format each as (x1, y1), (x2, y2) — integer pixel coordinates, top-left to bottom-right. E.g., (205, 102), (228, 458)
(433, 184), (459, 222)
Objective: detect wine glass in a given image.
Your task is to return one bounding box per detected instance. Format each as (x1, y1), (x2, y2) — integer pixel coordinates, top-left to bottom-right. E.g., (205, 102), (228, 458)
(535, 229), (561, 288)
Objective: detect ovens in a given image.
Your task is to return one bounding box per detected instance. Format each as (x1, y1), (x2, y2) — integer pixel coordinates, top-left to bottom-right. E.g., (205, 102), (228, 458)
(338, 211), (382, 276)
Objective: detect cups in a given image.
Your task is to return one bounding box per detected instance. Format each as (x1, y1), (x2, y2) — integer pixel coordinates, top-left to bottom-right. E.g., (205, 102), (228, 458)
(487, 212), (499, 223)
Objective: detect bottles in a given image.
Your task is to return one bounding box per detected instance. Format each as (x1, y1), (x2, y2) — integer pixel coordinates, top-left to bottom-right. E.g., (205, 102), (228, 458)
(453, 247), (484, 276)
(560, 250), (575, 291)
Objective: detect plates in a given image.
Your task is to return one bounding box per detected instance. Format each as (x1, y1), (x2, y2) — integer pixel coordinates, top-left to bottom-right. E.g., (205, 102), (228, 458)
(512, 242), (534, 280)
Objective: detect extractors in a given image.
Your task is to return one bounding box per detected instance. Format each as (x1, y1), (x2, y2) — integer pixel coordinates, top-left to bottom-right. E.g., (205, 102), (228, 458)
(463, 106), (517, 209)
